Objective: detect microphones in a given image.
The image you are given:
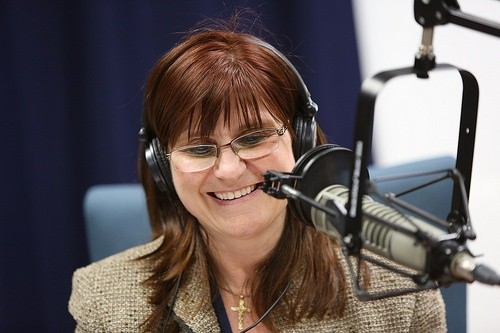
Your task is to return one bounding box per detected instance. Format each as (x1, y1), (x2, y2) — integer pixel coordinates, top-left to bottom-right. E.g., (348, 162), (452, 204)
(263, 144), (499, 291)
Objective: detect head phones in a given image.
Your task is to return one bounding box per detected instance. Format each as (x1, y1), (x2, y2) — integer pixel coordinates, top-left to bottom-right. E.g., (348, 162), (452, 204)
(140, 33), (317, 194)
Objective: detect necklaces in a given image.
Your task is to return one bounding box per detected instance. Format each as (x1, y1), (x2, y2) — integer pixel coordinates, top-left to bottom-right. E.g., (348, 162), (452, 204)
(222, 276), (258, 330)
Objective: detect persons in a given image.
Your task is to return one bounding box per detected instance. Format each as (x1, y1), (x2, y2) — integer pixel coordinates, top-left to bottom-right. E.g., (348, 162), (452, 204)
(69, 28), (449, 332)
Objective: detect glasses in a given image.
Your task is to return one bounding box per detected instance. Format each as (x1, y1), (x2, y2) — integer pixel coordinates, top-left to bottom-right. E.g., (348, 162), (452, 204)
(165, 121), (288, 175)
